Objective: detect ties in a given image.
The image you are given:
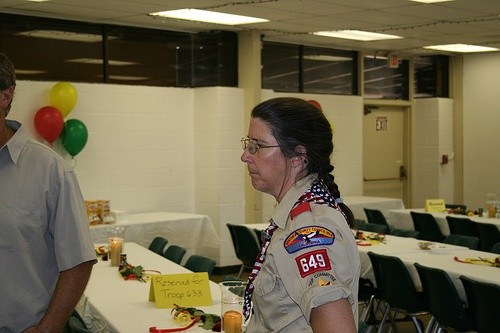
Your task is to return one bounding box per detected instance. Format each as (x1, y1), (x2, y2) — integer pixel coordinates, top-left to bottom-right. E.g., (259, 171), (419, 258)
(243, 177), (347, 324)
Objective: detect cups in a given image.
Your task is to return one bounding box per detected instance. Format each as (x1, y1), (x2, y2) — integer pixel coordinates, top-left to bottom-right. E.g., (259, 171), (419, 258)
(219, 280), (247, 333)
(84, 199), (111, 226)
(486, 192), (497, 219)
(107, 226), (126, 267)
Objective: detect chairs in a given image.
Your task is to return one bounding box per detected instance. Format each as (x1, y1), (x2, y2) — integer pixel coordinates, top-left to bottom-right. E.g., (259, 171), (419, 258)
(148, 224), (261, 291)
(68, 315), (92, 333)
(353, 208), (500, 333)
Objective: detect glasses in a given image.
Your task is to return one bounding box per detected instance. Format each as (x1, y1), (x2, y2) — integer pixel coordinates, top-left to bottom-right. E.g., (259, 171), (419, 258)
(241, 139), (292, 155)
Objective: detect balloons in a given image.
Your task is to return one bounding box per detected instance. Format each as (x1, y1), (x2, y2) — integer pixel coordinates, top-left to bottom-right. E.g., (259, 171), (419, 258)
(60, 119), (88, 156)
(34, 106), (64, 143)
(49, 82), (78, 117)
(308, 100), (335, 134)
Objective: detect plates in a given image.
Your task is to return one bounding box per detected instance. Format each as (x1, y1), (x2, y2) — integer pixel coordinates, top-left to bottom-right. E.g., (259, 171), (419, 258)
(419, 242), (434, 250)
(428, 244), (450, 253)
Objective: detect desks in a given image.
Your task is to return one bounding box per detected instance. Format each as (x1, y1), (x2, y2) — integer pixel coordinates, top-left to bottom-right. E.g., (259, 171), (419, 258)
(350, 228), (500, 303)
(76, 241), (252, 333)
(389, 207), (500, 239)
(90, 211), (221, 260)
(342, 197), (404, 226)
(248, 222), (269, 231)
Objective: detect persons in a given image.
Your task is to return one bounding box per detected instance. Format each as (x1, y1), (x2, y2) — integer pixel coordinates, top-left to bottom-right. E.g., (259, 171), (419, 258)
(241, 97), (361, 333)
(0, 53), (98, 333)
